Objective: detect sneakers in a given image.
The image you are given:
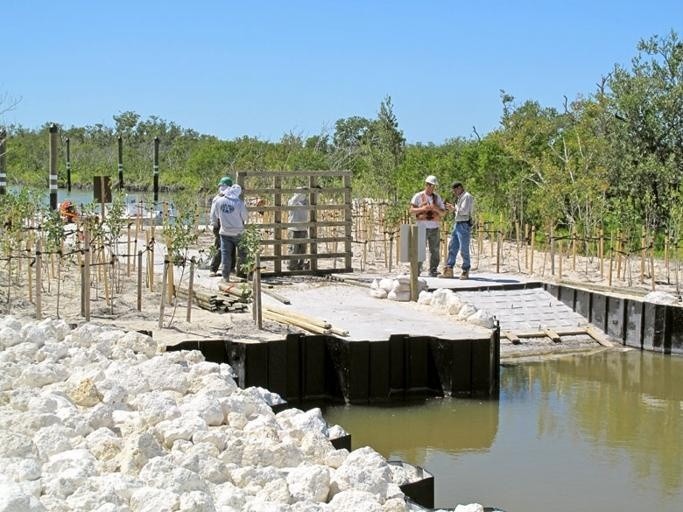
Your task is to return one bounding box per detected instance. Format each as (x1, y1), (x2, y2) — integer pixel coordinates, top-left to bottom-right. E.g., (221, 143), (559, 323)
(430, 268), (441, 277)
(209, 268), (218, 277)
(220, 277), (229, 283)
(287, 263), (308, 272)
(236, 272), (247, 278)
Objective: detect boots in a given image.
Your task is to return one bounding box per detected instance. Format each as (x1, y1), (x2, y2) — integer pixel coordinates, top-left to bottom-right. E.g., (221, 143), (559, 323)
(459, 270), (469, 280)
(437, 266), (454, 278)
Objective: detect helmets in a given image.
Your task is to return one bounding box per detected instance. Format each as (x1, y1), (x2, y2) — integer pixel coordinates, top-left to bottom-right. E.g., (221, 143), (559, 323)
(218, 177), (232, 187)
(426, 175), (439, 186)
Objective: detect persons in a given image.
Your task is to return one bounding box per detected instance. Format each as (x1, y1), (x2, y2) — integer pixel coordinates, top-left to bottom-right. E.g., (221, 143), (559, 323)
(288, 186), (311, 270)
(409, 175), (473, 280)
(210, 177), (248, 282)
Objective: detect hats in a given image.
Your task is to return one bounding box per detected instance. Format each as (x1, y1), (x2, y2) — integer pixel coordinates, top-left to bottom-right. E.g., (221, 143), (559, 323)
(451, 182), (462, 189)
